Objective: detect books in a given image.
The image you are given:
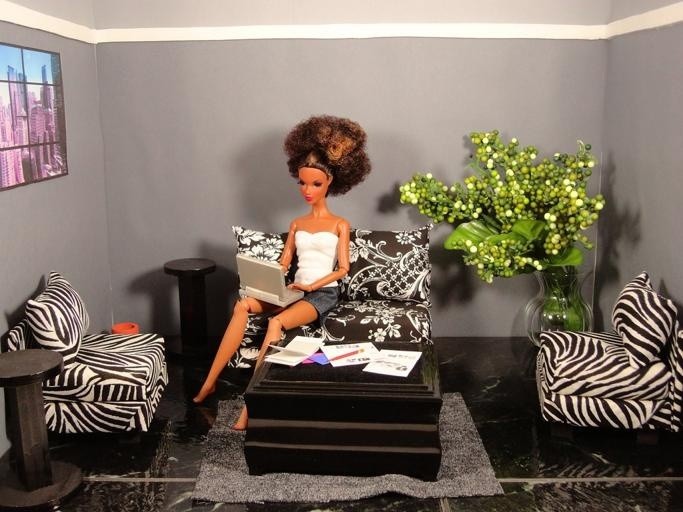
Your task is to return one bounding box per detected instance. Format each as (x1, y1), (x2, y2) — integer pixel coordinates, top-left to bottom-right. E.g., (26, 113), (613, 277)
(261, 335), (325, 369)
(360, 349), (423, 378)
(318, 341), (380, 368)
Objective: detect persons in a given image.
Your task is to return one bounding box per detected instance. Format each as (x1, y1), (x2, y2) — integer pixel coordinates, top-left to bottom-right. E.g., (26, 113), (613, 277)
(188, 111), (372, 433)
